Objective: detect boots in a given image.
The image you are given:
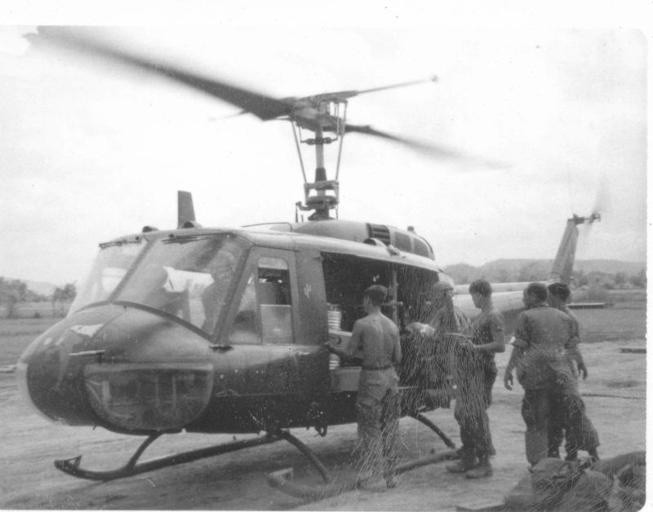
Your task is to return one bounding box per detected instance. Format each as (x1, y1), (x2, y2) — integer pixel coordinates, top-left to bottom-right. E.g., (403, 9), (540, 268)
(445, 445), (477, 474)
(463, 452), (494, 481)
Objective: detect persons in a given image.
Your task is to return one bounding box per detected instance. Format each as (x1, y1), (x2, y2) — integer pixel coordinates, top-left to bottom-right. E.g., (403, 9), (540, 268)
(504, 281), (600, 475)
(445, 279), (505, 480)
(545, 283), (589, 469)
(330, 285), (404, 493)
(199, 251), (261, 347)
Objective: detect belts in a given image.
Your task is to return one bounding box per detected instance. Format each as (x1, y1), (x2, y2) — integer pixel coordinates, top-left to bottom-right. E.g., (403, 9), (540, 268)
(361, 362), (393, 372)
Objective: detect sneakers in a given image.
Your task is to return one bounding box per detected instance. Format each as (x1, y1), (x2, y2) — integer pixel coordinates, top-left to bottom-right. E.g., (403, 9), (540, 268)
(359, 475), (402, 493)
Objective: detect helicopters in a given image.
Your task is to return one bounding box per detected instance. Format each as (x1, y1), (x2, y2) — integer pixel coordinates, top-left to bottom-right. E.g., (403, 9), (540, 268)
(15, 26), (606, 498)
